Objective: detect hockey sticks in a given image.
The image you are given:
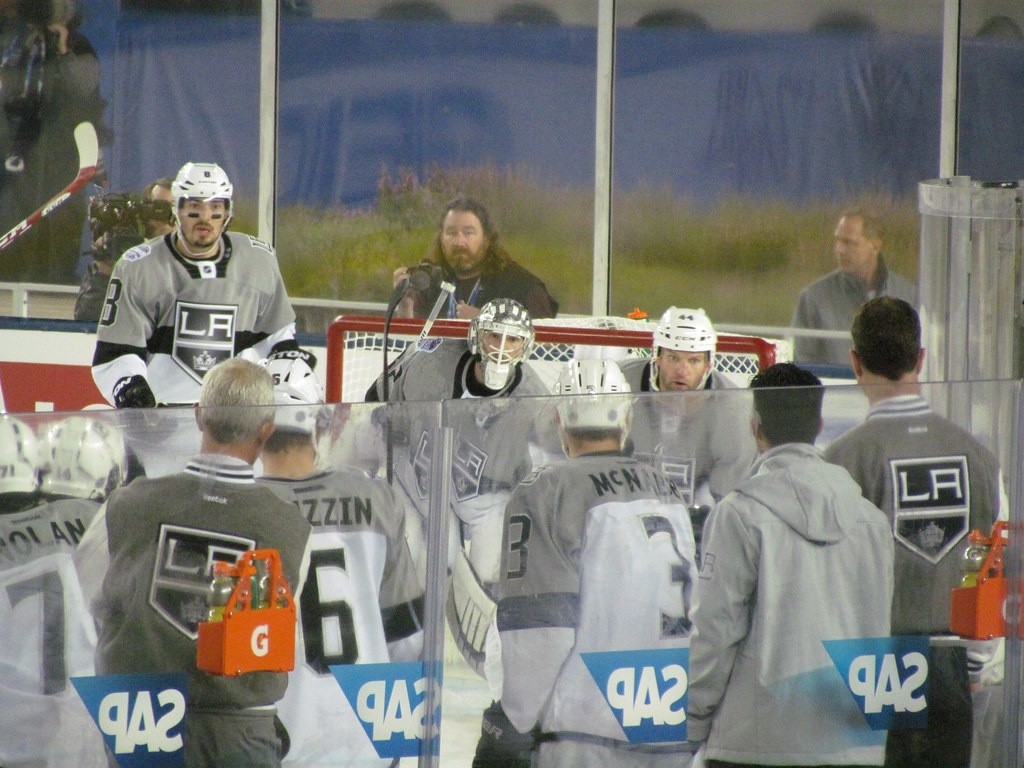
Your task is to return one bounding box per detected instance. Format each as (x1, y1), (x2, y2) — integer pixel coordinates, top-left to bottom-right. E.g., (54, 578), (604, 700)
(418, 281), (457, 339)
(381, 276), (410, 485)
(0, 120), (100, 251)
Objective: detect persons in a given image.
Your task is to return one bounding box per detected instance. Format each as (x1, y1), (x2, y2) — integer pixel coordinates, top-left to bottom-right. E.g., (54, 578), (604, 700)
(824, 294), (1009, 768)
(617, 305), (761, 509)
(792, 207), (918, 366)
(0, 23), (109, 286)
(262, 357), (426, 768)
(686, 362), (895, 768)
(355, 298), (564, 677)
(74, 356), (314, 768)
(73, 177), (177, 321)
(471, 357), (699, 768)
(91, 162), (297, 409)
(393, 197), (558, 321)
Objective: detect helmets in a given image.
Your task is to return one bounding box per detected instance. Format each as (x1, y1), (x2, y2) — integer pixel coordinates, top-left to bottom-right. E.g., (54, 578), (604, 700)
(554, 357), (632, 447)
(39, 414), (120, 502)
(83, 405), (129, 483)
(259, 358), (329, 448)
(0, 414), (39, 496)
(650, 304), (716, 393)
(468, 296), (536, 389)
(171, 162), (234, 219)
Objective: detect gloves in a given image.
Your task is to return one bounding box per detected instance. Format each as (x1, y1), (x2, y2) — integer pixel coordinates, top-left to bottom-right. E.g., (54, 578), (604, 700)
(269, 351), (317, 374)
(472, 699), (541, 768)
(112, 376), (178, 443)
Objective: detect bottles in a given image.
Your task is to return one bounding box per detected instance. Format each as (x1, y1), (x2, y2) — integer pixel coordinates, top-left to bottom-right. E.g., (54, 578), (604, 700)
(960, 527), (990, 589)
(628, 307), (649, 322)
(993, 536), (1010, 580)
(207, 561), (240, 624)
(257, 559), (292, 610)
(238, 565), (262, 610)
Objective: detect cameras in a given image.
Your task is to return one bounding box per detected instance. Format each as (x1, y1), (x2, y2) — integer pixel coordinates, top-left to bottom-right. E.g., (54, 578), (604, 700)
(403, 258), (447, 292)
